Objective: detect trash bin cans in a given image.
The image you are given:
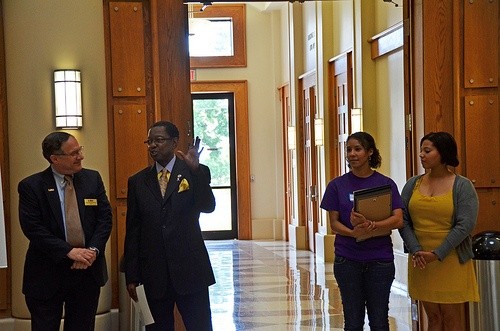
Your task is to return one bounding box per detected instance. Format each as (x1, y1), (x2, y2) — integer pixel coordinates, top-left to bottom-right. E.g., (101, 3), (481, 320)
(468, 231), (500, 331)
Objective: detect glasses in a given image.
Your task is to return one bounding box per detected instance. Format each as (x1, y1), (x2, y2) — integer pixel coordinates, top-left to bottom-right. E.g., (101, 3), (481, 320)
(143, 136), (174, 143)
(52, 146), (85, 158)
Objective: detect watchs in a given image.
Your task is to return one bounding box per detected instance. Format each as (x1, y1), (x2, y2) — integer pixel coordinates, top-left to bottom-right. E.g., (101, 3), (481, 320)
(89, 246), (98, 254)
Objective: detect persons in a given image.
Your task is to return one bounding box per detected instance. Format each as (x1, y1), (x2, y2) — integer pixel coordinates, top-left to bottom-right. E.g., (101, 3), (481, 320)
(373, 221), (376, 230)
(320, 132), (406, 331)
(398, 131), (481, 331)
(17, 131), (113, 331)
(124, 121), (216, 331)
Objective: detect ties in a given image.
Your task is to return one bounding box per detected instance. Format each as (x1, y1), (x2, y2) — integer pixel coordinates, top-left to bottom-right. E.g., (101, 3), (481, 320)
(159, 168), (169, 199)
(62, 175), (86, 249)
